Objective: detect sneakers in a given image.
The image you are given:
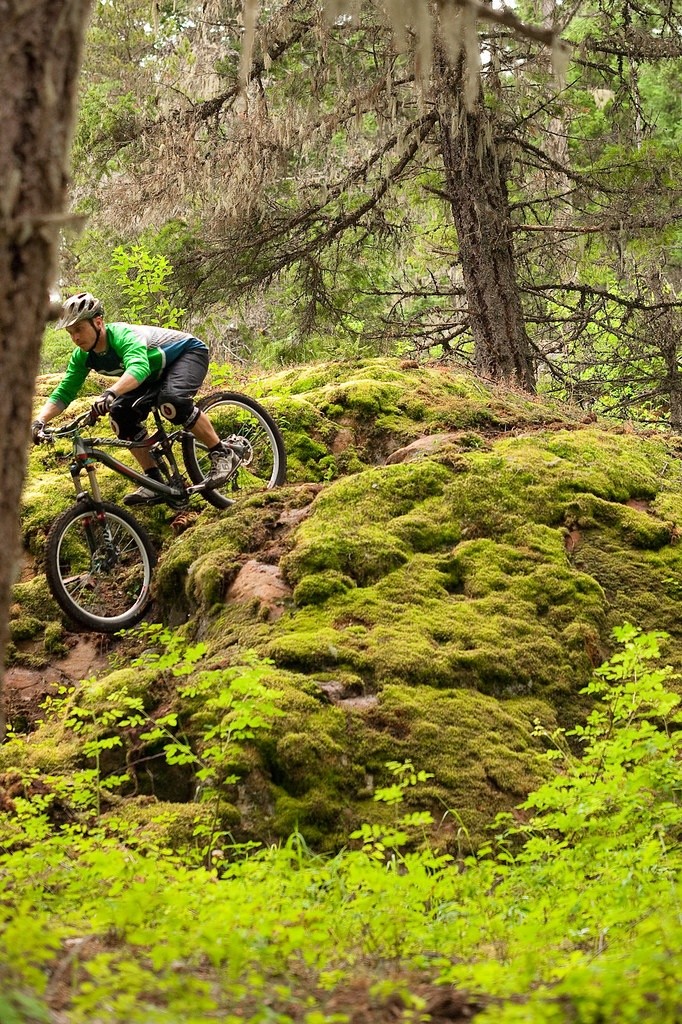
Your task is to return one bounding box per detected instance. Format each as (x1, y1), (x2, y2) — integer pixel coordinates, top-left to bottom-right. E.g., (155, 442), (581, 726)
(204, 449), (245, 491)
(123, 474), (169, 506)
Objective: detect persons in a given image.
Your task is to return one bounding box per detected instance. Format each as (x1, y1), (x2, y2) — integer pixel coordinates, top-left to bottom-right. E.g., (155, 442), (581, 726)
(29, 292), (240, 504)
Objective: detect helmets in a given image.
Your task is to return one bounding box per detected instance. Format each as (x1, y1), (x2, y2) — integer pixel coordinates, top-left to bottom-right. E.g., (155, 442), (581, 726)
(53, 291), (106, 332)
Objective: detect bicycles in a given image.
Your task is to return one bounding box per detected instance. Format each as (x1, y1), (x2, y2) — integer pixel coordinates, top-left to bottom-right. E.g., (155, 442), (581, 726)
(35, 379), (285, 629)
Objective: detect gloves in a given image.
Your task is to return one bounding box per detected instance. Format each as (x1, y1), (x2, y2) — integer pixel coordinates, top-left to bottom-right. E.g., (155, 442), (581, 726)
(30, 419), (46, 446)
(89, 388), (119, 421)
